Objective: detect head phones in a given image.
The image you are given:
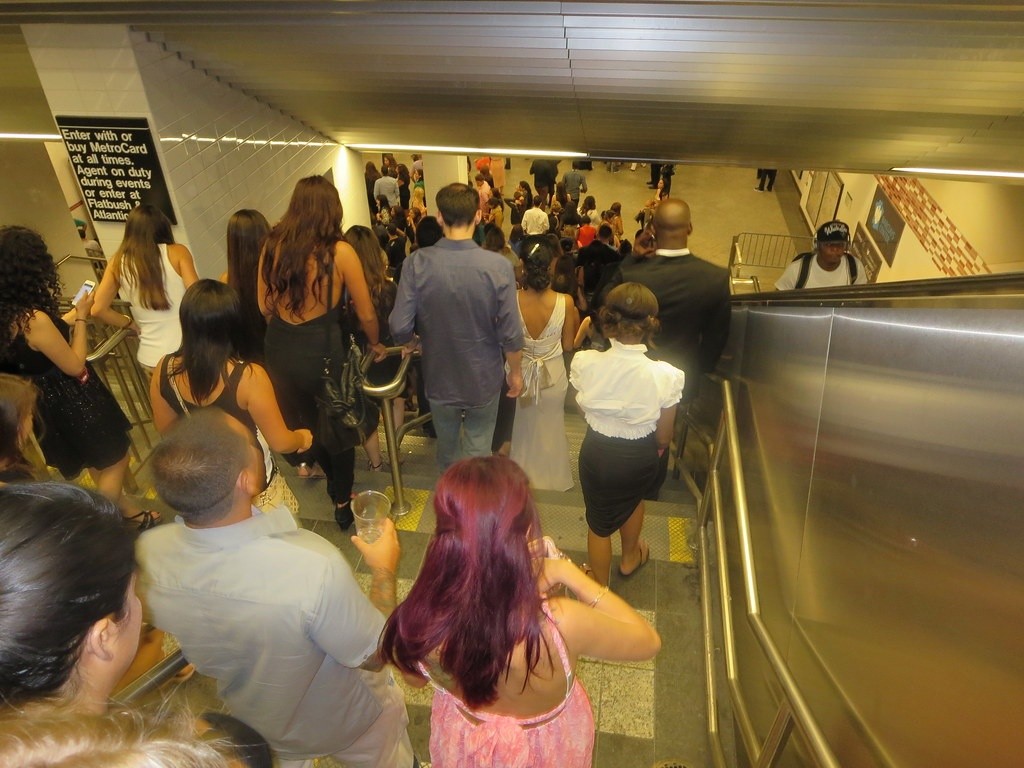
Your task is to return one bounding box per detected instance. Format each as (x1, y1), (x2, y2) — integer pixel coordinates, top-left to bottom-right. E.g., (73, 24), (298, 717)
(811, 231), (851, 251)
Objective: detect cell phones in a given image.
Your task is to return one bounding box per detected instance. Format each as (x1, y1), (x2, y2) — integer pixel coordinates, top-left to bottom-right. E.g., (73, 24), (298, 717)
(71, 279), (96, 306)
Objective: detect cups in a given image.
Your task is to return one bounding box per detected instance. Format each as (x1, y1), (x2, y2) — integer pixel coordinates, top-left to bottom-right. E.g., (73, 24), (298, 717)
(350, 490), (392, 545)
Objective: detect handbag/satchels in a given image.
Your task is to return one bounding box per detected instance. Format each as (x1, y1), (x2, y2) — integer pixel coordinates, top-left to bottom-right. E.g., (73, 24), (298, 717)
(317, 344), (382, 446)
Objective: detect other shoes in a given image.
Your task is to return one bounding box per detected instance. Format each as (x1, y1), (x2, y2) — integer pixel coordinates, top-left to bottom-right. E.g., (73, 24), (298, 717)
(367, 458), (382, 472)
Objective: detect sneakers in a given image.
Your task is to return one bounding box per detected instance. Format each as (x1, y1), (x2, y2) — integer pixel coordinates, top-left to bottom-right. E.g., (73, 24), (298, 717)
(754, 186), (763, 192)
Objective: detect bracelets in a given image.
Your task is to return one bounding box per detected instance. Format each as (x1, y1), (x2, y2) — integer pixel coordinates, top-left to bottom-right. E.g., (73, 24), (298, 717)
(589, 585), (613, 608)
(368, 340), (381, 345)
(75, 318), (88, 323)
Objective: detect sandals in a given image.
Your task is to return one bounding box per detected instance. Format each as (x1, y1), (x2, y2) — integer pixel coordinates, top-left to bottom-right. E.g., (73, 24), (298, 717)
(295, 462), (326, 478)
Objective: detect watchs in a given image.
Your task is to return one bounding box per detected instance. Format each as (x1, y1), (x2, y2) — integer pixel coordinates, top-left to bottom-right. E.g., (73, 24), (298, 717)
(123, 314), (132, 329)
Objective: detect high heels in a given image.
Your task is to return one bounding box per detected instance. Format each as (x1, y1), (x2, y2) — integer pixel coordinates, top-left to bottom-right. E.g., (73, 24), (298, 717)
(334, 500), (354, 529)
(122, 509), (161, 530)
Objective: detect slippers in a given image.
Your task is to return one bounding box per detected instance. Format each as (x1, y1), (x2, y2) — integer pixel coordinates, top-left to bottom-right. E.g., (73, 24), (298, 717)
(618, 546), (652, 579)
(580, 563), (594, 577)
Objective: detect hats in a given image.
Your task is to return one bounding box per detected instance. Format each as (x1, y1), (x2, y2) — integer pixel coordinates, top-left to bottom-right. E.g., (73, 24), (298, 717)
(817, 221), (850, 241)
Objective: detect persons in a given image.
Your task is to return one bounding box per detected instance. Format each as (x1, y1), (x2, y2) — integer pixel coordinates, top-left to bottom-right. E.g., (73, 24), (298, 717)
(256, 174), (379, 532)
(149, 278), (314, 515)
(381, 457), (663, 768)
(755, 168), (777, 191)
(216, 208), (271, 361)
(129, 406), (417, 768)
(504, 234), (574, 493)
(590, 198), (731, 500)
(91, 203), (199, 386)
(568, 283), (685, 588)
(345, 152), (674, 470)
(389, 181), (525, 472)
(0, 374), (66, 485)
(0, 481), (273, 768)
(0, 224), (161, 532)
(774, 221), (868, 291)
